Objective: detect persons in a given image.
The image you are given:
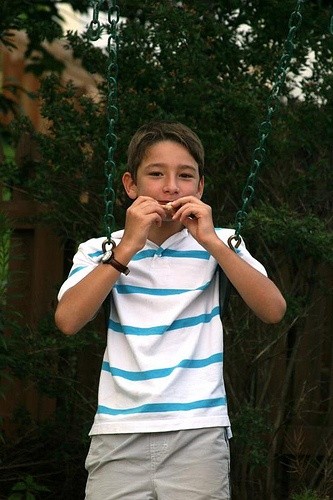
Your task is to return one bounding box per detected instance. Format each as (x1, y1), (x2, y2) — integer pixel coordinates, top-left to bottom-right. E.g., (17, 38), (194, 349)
(54, 123), (285, 500)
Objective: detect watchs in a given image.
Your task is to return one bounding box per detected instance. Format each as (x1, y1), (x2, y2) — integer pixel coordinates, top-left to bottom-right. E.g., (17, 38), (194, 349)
(98, 249), (131, 276)
